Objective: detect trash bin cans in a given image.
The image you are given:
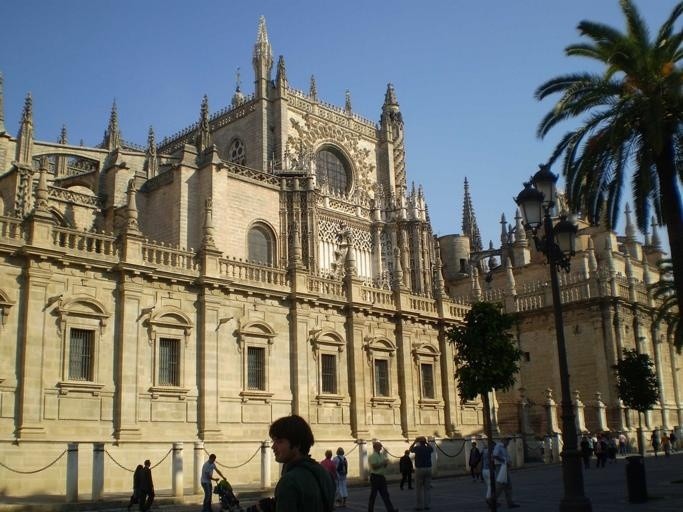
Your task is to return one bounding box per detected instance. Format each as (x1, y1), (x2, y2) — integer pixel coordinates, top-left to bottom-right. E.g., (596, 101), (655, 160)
(624, 456), (648, 504)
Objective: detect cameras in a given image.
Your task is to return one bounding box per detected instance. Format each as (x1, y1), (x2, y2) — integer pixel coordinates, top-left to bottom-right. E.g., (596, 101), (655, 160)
(240, 496), (276, 512)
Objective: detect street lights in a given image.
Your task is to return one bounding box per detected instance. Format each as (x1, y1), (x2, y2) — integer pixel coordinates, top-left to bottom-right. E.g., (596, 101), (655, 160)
(513, 164), (593, 512)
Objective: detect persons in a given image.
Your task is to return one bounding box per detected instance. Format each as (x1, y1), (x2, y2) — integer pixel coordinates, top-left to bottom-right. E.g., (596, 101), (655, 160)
(469, 441), (479, 481)
(138, 460), (154, 511)
(491, 436), (520, 509)
(366, 442), (398, 512)
(523, 427), (678, 469)
(199, 454), (226, 511)
(268, 415), (336, 511)
(399, 449), (412, 490)
(479, 440), (495, 505)
(126, 463), (142, 511)
(410, 435), (433, 509)
(331, 446), (348, 508)
(319, 449), (336, 476)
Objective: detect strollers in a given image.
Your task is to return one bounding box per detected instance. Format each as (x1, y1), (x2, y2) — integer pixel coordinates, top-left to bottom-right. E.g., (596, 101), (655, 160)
(213, 478), (245, 512)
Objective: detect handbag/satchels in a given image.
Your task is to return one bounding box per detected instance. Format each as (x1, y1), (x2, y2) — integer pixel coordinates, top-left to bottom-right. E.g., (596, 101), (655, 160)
(496, 465), (508, 483)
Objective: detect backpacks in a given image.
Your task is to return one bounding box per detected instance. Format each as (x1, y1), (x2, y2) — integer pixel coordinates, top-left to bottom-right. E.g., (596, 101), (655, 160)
(337, 456), (347, 476)
